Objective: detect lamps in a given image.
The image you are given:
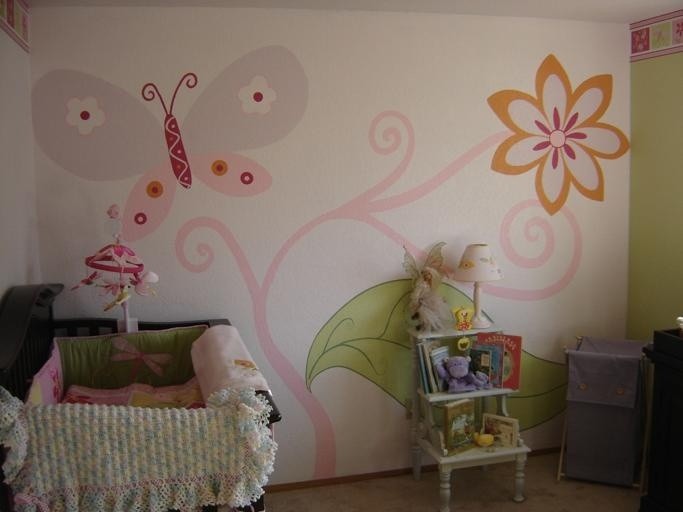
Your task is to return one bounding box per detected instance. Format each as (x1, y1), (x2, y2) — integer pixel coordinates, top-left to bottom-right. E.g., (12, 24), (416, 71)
(452, 242), (504, 328)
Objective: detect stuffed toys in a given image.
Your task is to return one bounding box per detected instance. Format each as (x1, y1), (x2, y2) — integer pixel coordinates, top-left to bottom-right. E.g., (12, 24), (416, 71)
(435, 355), (494, 392)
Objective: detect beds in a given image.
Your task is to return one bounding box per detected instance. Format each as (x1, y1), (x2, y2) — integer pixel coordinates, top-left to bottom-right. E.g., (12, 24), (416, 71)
(0, 282), (281, 511)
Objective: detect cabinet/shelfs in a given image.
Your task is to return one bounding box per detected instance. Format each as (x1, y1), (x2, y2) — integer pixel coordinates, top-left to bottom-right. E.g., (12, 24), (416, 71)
(404, 323), (532, 511)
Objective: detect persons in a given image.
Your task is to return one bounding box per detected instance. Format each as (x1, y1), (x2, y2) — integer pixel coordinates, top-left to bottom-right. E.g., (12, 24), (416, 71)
(402, 242), (473, 334)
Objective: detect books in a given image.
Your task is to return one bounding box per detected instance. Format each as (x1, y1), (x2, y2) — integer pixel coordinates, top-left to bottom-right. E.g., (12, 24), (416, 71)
(416, 340), (449, 394)
(483, 414), (519, 448)
(469, 332), (522, 390)
(447, 398), (475, 447)
